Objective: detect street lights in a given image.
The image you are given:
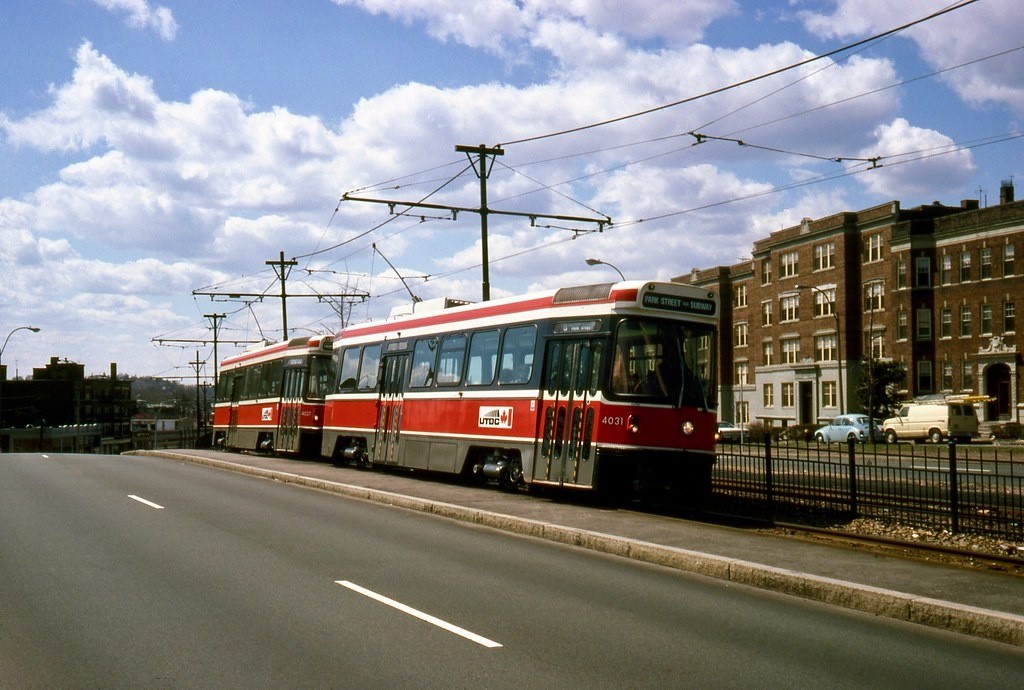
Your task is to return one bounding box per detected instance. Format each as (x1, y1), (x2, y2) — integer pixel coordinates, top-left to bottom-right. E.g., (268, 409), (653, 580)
(795, 285), (844, 417)
(0, 326), (41, 364)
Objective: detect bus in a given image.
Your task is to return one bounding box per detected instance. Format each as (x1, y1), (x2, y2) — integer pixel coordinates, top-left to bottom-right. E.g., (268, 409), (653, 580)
(212, 336), (335, 457)
(322, 278), (720, 499)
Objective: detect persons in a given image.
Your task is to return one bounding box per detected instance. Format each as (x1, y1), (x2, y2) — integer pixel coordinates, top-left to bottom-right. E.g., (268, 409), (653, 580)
(515, 365), (530, 383)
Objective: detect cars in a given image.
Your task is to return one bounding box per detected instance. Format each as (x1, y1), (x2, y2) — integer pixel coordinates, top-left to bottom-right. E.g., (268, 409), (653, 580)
(813, 415), (881, 443)
(718, 421), (750, 443)
(873, 418), (885, 437)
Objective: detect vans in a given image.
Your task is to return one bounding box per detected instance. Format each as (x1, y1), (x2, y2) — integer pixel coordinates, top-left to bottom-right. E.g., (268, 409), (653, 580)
(882, 403), (979, 443)
